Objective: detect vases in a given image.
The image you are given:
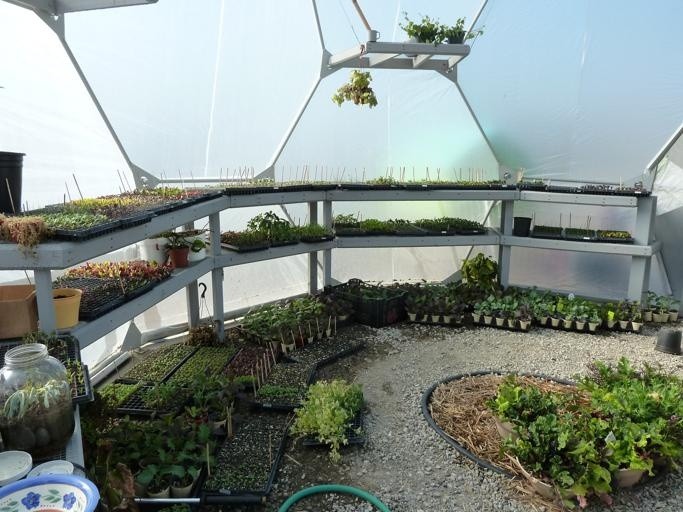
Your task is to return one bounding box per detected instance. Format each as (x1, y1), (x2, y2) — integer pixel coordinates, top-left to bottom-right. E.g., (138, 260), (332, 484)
(0, 150), (26, 215)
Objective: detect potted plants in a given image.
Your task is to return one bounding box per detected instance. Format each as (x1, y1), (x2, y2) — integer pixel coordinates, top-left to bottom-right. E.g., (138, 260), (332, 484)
(328, 278), (682, 335)
(1, 380), (367, 511)
(399, 12), (439, 57)
(487, 354), (682, 511)
(440, 12), (486, 44)
(331, 69), (378, 108)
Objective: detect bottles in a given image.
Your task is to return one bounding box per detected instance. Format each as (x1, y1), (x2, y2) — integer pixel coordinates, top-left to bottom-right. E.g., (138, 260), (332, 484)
(0, 340), (75, 458)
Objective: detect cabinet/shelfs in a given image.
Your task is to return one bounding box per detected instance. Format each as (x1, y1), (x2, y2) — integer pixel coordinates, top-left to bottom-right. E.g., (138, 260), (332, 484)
(1, 181), (336, 478)
(331, 180), (661, 310)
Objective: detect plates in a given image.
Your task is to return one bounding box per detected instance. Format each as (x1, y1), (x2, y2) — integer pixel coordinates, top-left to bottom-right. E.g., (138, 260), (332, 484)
(25, 459), (75, 479)
(0, 450), (33, 487)
(0, 472), (101, 512)
